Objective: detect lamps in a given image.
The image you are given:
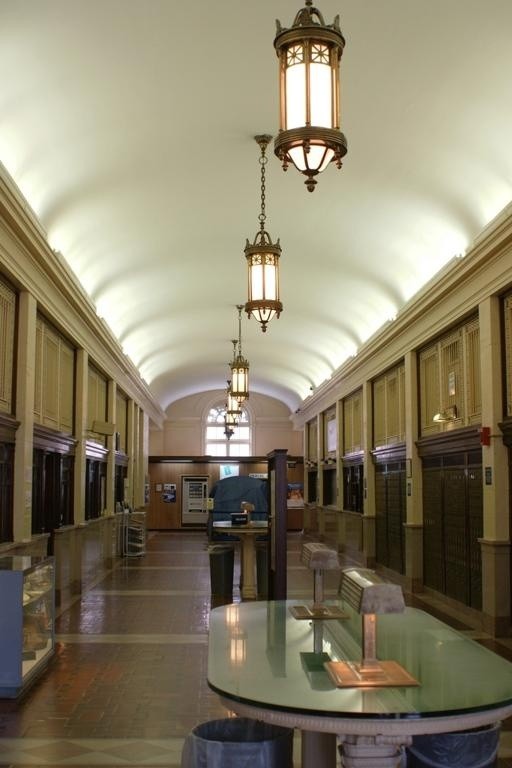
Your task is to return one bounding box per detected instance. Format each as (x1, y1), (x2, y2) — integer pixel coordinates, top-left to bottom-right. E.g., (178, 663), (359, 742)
(240, 502), (249, 513)
(323, 567), (426, 688)
(273, 1), (350, 197)
(242, 131), (286, 334)
(229, 631), (247, 663)
(289, 543), (351, 619)
(240, 504), (254, 529)
(227, 303), (252, 404)
(222, 336), (245, 442)
(300, 618), (336, 691)
(433, 413), (462, 423)
(225, 603), (239, 630)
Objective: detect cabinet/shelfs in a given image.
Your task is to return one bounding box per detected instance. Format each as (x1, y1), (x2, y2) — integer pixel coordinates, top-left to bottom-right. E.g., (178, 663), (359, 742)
(0, 554), (56, 699)
(122, 512), (147, 560)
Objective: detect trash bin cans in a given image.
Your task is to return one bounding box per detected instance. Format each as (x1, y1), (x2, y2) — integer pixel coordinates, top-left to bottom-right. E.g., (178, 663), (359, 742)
(188, 716), (294, 768)
(208, 545), (234, 594)
(405, 718), (502, 768)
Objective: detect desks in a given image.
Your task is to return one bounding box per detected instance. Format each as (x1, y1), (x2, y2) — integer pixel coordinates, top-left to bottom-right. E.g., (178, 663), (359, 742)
(206, 599), (511, 767)
(213, 520), (268, 598)
